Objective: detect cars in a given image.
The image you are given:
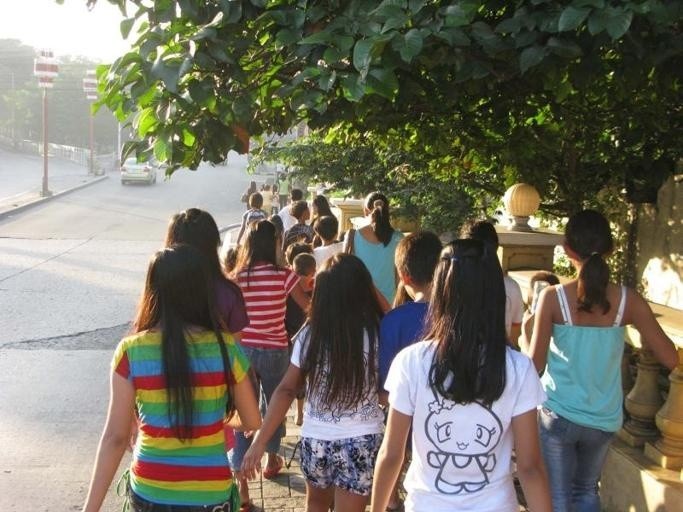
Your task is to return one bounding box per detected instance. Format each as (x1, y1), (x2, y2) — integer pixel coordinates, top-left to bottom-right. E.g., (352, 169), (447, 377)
(121, 157), (156, 185)
